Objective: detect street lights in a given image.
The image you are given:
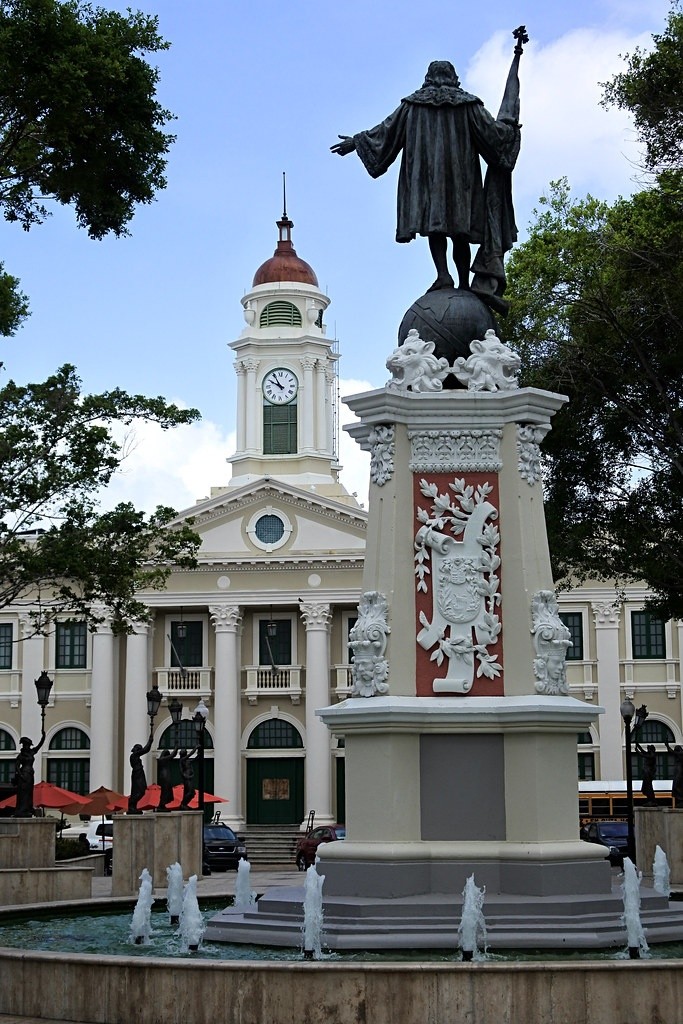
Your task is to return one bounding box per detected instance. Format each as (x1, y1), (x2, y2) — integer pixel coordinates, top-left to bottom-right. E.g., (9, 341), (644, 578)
(193, 699), (209, 821)
(620, 697), (635, 867)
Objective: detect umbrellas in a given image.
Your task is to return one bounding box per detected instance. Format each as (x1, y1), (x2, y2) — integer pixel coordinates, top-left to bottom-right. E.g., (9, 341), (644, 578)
(0, 781), (229, 851)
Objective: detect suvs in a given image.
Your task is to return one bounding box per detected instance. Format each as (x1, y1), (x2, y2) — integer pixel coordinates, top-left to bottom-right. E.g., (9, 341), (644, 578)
(87, 820), (114, 872)
(203, 822), (247, 873)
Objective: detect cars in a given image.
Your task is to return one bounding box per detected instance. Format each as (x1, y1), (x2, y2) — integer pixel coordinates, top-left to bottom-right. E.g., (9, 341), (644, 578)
(295, 825), (344, 872)
(582, 821), (630, 867)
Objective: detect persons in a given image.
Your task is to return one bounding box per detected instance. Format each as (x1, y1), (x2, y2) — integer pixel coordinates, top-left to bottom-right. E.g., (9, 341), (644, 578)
(329, 62), (521, 293)
(180, 745), (199, 810)
(15, 730), (45, 817)
(636, 744), (656, 806)
(157, 750), (177, 813)
(128, 734), (154, 816)
(665, 737), (683, 808)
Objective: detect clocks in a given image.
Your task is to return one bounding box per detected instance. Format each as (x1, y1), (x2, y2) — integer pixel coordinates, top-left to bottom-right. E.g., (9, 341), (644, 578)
(262, 367), (298, 405)
